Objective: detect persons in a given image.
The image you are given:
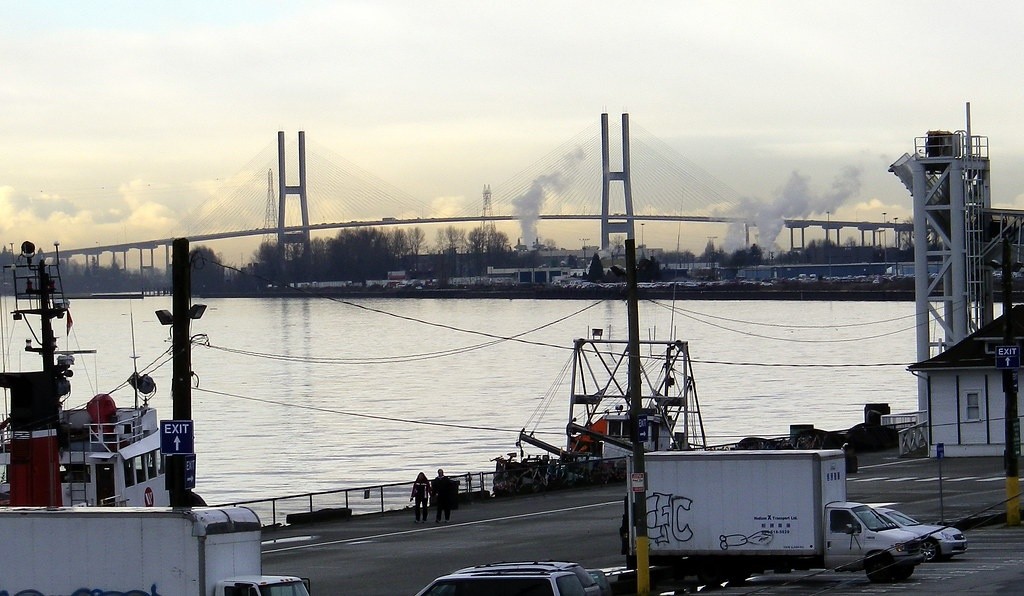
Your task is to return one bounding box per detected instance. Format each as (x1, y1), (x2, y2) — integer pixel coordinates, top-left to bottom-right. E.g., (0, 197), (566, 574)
(410, 472), (431, 524)
(432, 469), (452, 523)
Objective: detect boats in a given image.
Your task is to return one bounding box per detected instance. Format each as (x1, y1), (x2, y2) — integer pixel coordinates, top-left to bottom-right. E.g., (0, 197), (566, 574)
(0, 240), (173, 507)
(515, 324), (710, 463)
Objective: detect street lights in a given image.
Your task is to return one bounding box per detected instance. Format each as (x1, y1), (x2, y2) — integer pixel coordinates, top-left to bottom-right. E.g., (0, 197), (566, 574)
(707, 235), (717, 257)
(826, 211), (832, 280)
(894, 216), (899, 275)
(580, 238), (592, 272)
(641, 223), (646, 257)
(874, 230), (885, 248)
(882, 211), (888, 278)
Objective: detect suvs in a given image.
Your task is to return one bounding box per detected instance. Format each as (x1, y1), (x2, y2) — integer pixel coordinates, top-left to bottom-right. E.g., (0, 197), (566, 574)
(411, 559), (613, 596)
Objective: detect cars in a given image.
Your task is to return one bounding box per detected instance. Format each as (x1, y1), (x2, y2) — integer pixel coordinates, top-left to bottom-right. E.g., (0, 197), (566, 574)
(551, 270), (1024, 288)
(855, 508), (968, 563)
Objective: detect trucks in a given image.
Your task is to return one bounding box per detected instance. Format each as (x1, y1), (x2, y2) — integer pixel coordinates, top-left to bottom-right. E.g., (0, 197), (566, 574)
(0, 500), (316, 596)
(626, 447), (924, 582)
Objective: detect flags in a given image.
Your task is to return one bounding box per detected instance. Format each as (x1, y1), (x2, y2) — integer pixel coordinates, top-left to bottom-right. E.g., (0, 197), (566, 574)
(67, 310), (73, 336)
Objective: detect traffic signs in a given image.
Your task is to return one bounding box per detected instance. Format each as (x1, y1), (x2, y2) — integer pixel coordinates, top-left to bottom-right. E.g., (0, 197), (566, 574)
(995, 346), (1021, 370)
(159, 419), (194, 454)
